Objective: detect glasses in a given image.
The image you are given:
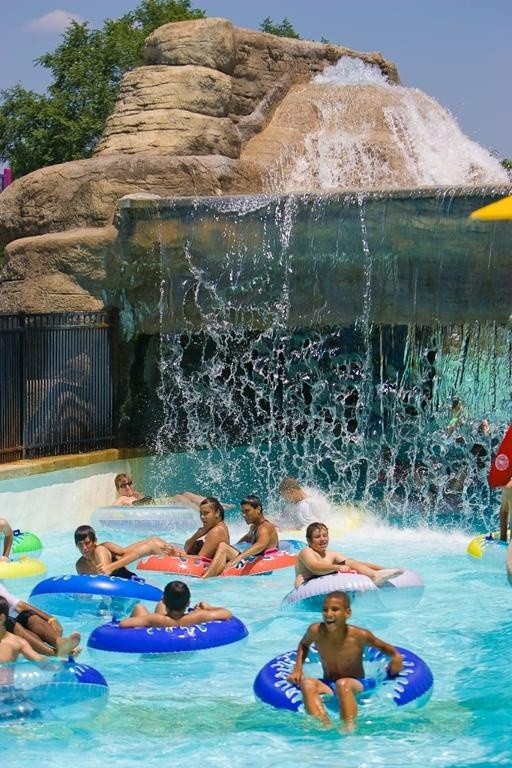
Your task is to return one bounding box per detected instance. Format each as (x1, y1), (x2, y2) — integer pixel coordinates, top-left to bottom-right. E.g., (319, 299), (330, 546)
(119, 480), (132, 489)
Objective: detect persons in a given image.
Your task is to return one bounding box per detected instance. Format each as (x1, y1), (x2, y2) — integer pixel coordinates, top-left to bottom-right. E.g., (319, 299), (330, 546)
(201, 493), (280, 581)
(0, 583), (83, 656)
(119, 580), (234, 629)
(497, 476), (512, 581)
(112, 473), (237, 514)
(1, 595), (60, 681)
(294, 522), (405, 590)
(0, 518), (15, 561)
(286, 590), (405, 734)
(376, 400), (505, 513)
(276, 477), (331, 532)
(102, 497), (231, 573)
(75, 526), (137, 580)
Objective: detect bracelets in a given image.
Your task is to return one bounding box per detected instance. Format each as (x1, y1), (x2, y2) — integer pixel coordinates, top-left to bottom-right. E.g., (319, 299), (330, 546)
(48, 616), (57, 625)
(179, 551), (182, 557)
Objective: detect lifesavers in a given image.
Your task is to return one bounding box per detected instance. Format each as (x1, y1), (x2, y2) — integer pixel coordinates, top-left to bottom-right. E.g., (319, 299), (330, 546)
(87, 612), (251, 660)
(0, 529), (43, 558)
(468, 530), (511, 570)
(278, 505), (365, 543)
(134, 539), (307, 577)
(281, 567), (426, 612)
(89, 505), (201, 533)
(251, 643), (434, 709)
(0, 660), (110, 721)
(27, 574), (163, 618)
(0, 559), (46, 583)
